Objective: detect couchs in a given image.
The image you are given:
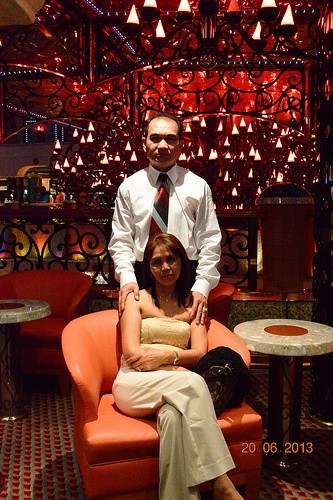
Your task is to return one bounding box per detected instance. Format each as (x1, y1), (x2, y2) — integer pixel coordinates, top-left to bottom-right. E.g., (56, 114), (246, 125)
(0, 270), (93, 397)
(62, 309), (262, 500)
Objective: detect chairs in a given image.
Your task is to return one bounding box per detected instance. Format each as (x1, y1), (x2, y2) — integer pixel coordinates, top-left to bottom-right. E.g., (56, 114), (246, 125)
(207, 282), (235, 329)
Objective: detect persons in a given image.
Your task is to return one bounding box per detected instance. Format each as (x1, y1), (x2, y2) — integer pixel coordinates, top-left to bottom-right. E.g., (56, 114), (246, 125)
(112, 234), (247, 500)
(106, 114), (223, 325)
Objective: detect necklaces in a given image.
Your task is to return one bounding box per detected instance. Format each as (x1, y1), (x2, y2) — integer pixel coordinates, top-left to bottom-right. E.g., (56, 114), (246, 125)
(156, 291), (174, 303)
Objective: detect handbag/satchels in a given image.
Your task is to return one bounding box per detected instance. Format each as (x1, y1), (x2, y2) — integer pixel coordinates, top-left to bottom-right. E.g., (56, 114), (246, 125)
(202, 363), (235, 415)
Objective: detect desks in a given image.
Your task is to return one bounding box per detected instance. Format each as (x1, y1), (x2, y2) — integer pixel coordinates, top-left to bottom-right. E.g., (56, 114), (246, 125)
(0, 299), (52, 421)
(234, 319), (333, 478)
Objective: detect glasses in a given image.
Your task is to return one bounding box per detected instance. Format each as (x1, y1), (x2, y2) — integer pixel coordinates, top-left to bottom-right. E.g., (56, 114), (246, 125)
(149, 172), (171, 245)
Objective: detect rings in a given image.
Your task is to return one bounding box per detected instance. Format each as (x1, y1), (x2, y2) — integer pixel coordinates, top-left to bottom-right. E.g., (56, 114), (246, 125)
(202, 307), (206, 313)
